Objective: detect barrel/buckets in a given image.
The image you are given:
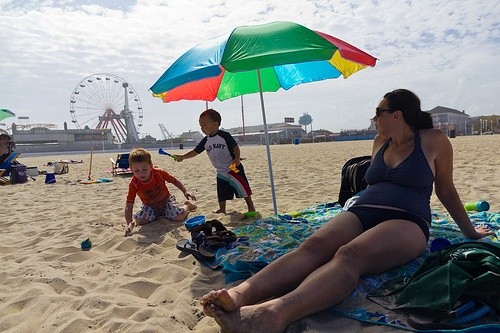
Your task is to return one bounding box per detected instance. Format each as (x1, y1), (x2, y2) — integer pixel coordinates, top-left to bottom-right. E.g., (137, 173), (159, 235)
(185, 215), (207, 229)
(45, 172), (56, 184)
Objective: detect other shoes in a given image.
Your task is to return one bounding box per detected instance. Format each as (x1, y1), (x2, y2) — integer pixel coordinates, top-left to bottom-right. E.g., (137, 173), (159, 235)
(175, 219), (237, 261)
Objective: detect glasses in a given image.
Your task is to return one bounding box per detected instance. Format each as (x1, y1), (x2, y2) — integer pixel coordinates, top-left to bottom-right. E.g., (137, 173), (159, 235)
(376, 107), (390, 117)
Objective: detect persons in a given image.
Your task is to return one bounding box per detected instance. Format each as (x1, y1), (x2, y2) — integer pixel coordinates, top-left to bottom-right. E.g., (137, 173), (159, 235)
(124, 148), (197, 237)
(0, 133), (11, 164)
(199, 89), (493, 333)
(174, 109), (256, 213)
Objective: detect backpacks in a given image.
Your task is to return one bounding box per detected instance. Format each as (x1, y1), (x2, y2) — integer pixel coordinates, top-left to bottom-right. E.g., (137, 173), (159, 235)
(116, 154), (128, 168)
(337, 156), (372, 208)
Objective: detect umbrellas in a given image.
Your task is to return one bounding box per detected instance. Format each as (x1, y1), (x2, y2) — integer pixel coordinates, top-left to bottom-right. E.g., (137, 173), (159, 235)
(147, 21), (380, 216)
(0, 107), (14, 122)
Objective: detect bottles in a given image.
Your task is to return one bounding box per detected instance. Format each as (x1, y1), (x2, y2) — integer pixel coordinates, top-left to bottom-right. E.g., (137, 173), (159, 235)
(465, 201), (490, 212)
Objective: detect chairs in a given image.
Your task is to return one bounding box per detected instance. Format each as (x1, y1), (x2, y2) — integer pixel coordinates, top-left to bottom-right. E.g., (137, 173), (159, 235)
(110, 153), (132, 175)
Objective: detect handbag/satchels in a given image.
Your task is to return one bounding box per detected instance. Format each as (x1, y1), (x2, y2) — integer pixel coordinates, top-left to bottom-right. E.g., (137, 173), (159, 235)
(45, 174), (55, 183)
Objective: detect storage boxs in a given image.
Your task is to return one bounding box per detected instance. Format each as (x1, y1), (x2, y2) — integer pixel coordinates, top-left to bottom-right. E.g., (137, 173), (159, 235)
(27, 166), (38, 176)
(9, 164), (28, 183)
